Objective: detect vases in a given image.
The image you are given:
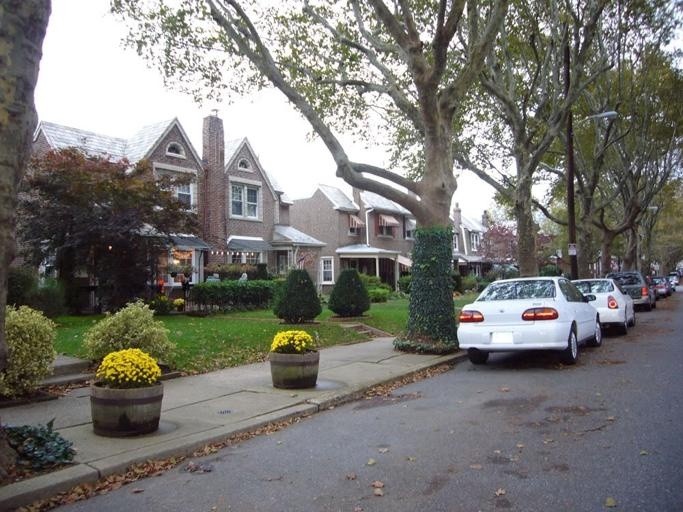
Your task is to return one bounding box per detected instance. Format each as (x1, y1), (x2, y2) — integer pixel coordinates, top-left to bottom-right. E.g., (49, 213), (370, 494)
(177, 305), (183, 311)
(90, 382), (164, 438)
(269, 349), (320, 389)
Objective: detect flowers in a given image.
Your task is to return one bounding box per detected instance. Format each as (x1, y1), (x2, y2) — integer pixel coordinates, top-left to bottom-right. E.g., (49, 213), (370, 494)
(173, 298), (185, 306)
(269, 329), (315, 355)
(94, 346), (161, 388)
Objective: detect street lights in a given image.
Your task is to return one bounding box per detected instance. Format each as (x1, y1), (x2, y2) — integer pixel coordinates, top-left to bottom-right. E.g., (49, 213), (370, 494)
(633, 205), (658, 271)
(567, 110), (618, 279)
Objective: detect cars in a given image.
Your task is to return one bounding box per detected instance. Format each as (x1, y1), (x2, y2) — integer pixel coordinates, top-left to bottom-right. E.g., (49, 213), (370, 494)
(456, 276), (603, 365)
(646, 266), (683, 298)
(571, 278), (635, 334)
(605, 270), (657, 312)
